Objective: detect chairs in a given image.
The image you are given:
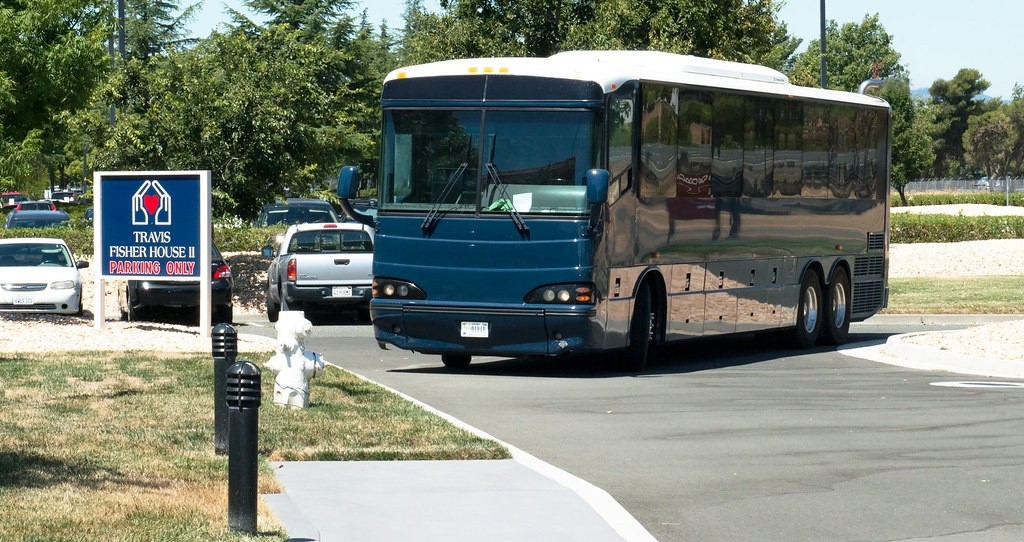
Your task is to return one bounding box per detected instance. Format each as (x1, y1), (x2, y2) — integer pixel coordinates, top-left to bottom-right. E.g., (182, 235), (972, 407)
(41, 251), (60, 265)
(296, 236), (316, 253)
(343, 234), (362, 252)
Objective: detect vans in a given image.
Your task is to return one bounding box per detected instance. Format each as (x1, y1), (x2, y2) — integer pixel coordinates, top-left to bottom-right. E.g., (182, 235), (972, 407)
(1, 192), (24, 206)
(17, 201), (57, 210)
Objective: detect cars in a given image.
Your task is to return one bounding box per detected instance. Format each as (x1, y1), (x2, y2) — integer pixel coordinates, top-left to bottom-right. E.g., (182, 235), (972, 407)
(118, 240), (233, 323)
(0, 238), (91, 317)
(253, 199), (336, 224)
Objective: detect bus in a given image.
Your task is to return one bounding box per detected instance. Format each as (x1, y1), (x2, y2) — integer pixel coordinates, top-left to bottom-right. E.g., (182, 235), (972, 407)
(334, 50), (893, 374)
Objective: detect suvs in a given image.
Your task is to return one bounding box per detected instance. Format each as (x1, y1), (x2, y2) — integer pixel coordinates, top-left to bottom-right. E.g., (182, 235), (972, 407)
(261, 223), (376, 322)
(3, 207), (70, 230)
(327, 196), (378, 220)
